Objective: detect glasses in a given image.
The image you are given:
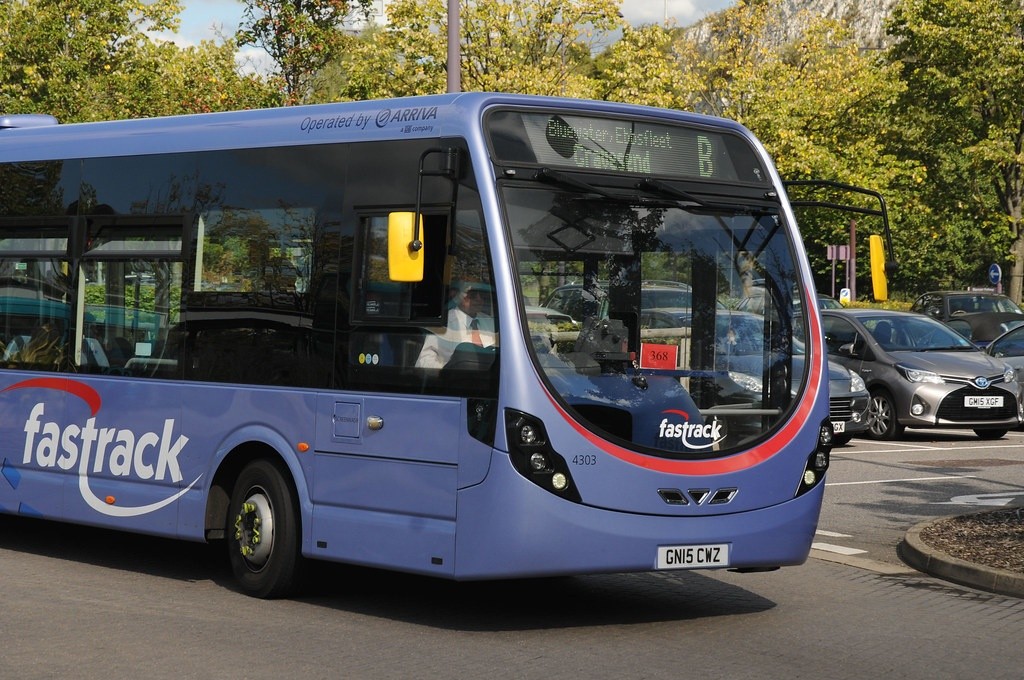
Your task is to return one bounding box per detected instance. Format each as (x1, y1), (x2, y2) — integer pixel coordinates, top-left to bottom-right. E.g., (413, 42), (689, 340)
(458, 287), (485, 299)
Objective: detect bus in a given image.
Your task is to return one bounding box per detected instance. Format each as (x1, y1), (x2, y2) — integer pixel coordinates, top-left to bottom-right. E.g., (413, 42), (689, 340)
(0, 90), (835, 600)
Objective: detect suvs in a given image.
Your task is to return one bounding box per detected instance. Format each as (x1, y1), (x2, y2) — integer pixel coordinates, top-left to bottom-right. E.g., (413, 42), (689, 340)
(540, 282), (730, 340)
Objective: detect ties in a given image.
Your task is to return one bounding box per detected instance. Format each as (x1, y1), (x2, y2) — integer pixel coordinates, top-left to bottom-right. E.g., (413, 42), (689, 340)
(470, 319), (484, 348)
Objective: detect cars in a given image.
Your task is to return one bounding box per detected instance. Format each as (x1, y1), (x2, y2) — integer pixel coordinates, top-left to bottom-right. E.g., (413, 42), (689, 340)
(981, 323), (1024, 432)
(909, 289), (1024, 350)
(733, 293), (845, 320)
(639, 308), (874, 447)
(787, 309), (1024, 439)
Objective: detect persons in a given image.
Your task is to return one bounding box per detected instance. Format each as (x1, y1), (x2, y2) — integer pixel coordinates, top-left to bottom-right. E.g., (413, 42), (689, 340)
(0, 270), (139, 373)
(416, 274), (499, 369)
